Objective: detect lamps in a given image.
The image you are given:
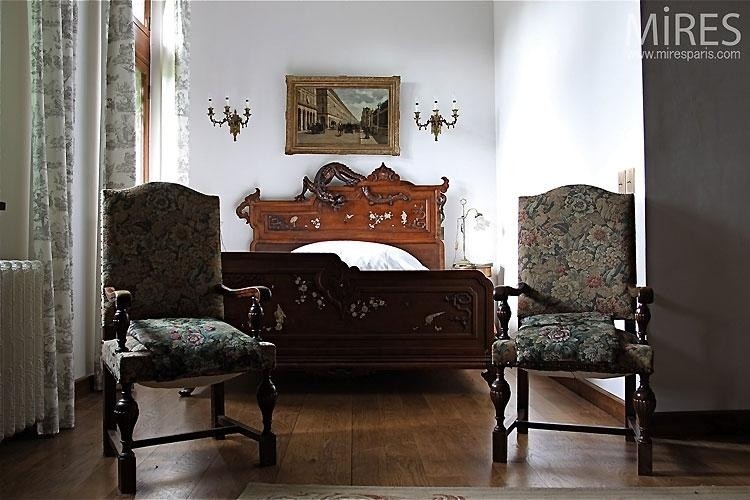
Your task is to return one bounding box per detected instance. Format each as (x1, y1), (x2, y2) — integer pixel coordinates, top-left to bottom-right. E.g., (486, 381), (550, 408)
(414, 97), (460, 139)
(452, 197), (482, 267)
(206, 94), (250, 141)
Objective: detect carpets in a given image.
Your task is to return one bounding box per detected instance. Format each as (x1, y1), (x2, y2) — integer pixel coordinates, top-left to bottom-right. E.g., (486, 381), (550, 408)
(237, 481), (749, 499)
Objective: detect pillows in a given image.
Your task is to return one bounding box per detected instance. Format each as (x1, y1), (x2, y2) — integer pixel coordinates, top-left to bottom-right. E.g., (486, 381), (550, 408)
(290, 240), (431, 272)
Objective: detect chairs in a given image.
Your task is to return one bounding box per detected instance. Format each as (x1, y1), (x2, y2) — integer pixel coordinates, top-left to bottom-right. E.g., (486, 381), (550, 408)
(483, 186), (657, 478)
(95, 181), (277, 494)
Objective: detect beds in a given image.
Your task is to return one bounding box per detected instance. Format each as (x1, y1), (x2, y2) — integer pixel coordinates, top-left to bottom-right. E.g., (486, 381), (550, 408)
(178, 162), (495, 399)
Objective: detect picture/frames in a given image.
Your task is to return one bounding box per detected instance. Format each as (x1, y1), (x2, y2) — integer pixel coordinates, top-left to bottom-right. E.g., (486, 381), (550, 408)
(282, 74), (402, 158)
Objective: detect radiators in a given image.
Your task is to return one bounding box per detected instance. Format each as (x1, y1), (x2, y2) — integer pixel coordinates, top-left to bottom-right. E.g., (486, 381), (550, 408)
(0, 259), (45, 440)
